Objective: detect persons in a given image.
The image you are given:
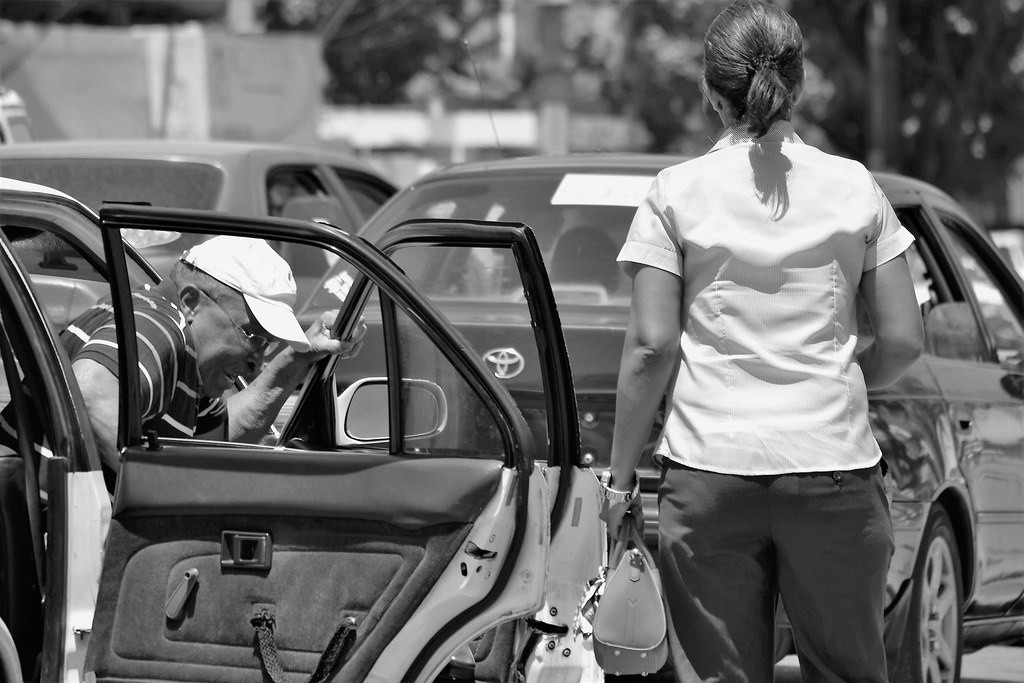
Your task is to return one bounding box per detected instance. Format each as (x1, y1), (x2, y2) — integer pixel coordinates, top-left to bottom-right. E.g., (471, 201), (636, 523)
(573, 0), (926, 683)
(0, 235), (368, 533)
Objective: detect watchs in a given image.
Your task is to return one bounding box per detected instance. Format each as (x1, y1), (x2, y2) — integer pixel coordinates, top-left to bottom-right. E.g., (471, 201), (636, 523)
(599, 470), (640, 503)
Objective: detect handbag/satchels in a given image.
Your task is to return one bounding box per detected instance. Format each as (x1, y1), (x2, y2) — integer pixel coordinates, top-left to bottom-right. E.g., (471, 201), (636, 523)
(592, 511), (669, 676)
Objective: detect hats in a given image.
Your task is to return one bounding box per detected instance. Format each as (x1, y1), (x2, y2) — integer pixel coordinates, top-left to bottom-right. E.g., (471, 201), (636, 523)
(179, 235), (312, 353)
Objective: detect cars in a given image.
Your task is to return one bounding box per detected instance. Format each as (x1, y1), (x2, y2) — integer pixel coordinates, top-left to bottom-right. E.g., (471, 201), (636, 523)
(1, 134), (1022, 682)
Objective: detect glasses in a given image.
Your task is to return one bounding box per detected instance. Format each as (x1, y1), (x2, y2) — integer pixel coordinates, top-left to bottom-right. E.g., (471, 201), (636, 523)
(200, 288), (271, 352)
